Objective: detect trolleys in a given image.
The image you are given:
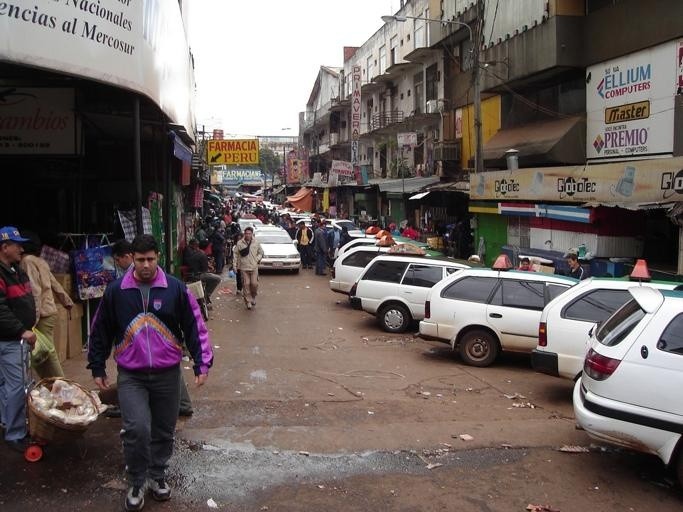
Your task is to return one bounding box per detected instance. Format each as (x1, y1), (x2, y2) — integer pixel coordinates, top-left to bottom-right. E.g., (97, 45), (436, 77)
(17, 337), (89, 464)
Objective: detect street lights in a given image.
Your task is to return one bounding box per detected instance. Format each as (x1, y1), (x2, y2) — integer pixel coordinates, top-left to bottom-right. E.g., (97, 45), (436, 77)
(381, 12), (483, 176)
(281, 127), (291, 175)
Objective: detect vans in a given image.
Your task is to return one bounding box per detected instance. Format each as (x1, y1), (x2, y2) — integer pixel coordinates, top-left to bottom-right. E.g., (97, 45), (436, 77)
(529, 260), (682, 382)
(337, 229), (410, 257)
(262, 200), (367, 237)
(349, 254), (472, 333)
(419, 254), (580, 367)
(329, 235), (431, 309)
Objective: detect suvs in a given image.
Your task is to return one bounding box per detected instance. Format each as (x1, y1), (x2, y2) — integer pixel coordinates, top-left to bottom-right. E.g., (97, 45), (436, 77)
(572, 288), (683, 477)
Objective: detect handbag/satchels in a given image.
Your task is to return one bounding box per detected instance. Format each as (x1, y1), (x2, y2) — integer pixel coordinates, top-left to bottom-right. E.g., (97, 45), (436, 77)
(114, 208), (153, 240)
(238, 239), (252, 257)
(68, 245), (126, 302)
(38, 244), (70, 277)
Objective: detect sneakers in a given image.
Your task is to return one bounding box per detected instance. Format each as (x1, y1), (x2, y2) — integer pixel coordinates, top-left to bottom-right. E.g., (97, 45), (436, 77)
(124, 482), (146, 511)
(246, 301), (253, 311)
(252, 299), (256, 305)
(148, 475), (171, 501)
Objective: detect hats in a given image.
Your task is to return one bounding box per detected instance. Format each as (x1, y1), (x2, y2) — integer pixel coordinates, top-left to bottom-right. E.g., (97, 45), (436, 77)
(0, 226), (30, 243)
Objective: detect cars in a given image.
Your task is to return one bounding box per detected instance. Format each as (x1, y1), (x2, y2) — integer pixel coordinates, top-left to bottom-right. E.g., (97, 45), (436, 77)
(253, 224), (301, 271)
(238, 214), (264, 233)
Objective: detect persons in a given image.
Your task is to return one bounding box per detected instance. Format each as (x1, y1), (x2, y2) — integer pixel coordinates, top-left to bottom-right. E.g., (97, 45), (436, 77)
(566, 253), (584, 279)
(86, 234), (214, 512)
(113, 238), (193, 418)
(181, 203), (351, 310)
(0, 226), (36, 453)
(18, 233), (73, 392)
(400, 219), (418, 240)
(517, 258), (536, 272)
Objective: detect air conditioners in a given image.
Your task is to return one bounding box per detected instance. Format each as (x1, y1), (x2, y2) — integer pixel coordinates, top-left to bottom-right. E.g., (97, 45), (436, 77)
(330, 133), (338, 145)
(426, 99), (442, 114)
